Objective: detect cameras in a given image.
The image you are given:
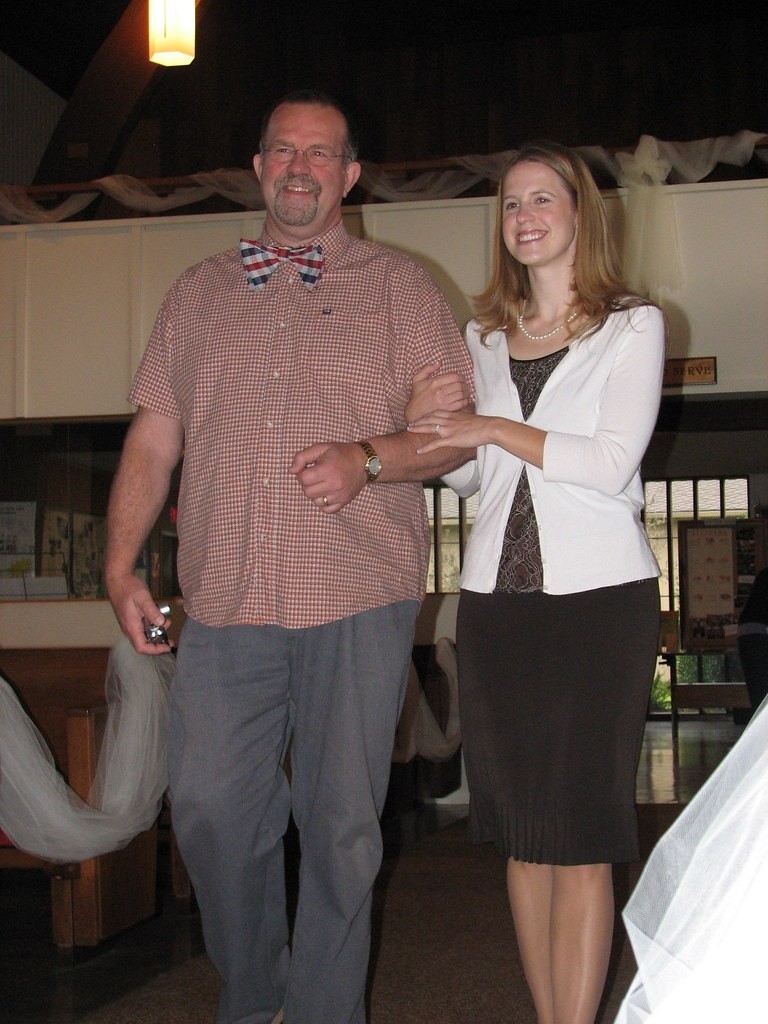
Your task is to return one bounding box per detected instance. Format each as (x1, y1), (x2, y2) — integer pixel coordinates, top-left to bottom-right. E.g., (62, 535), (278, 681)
(143, 602), (172, 645)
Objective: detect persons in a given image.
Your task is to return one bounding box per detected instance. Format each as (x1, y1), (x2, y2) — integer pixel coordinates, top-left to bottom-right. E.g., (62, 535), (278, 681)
(403, 142), (671, 1024)
(98, 87), (480, 1024)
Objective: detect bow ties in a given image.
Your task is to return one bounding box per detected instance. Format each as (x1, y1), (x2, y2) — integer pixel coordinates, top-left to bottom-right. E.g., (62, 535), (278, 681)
(239, 238), (326, 292)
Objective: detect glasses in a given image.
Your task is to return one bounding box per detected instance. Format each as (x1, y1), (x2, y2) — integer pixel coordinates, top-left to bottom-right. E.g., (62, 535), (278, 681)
(263, 140), (352, 168)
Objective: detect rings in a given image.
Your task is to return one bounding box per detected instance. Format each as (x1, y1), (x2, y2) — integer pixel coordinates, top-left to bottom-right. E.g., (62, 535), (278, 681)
(322, 496), (328, 506)
(434, 425), (440, 435)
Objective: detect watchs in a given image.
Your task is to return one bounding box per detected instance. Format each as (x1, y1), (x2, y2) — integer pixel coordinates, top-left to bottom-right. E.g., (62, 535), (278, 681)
(354, 438), (383, 484)
